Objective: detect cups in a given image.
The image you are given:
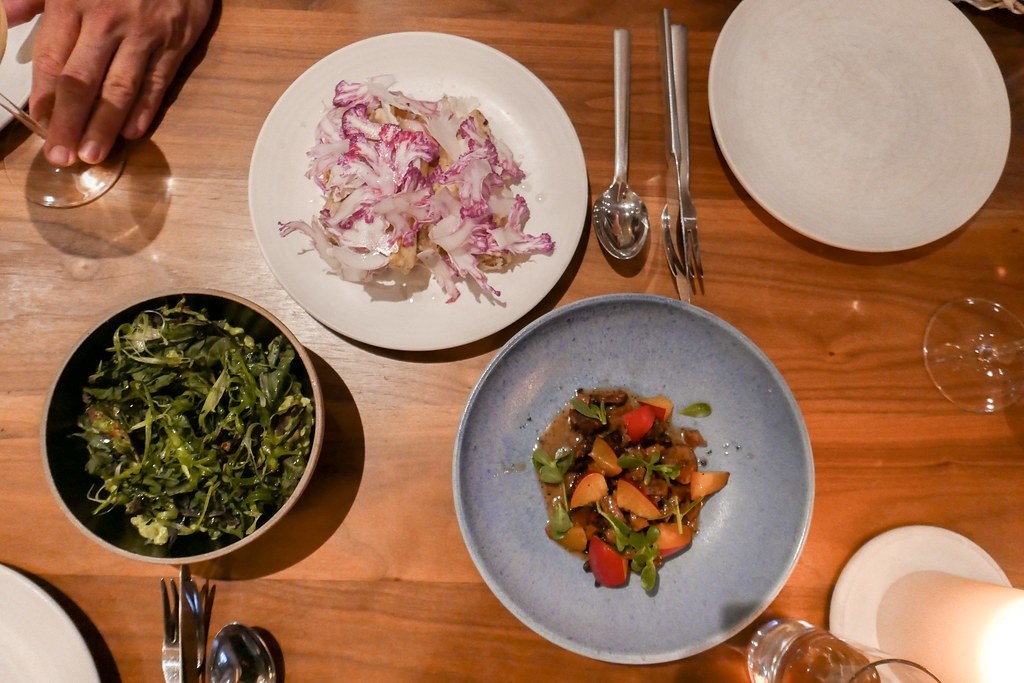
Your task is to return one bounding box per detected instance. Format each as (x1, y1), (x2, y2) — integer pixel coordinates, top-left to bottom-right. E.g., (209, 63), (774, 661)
(744, 618), (942, 683)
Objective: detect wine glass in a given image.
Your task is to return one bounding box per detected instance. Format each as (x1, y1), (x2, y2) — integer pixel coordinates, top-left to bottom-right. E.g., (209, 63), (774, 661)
(0, 0), (126, 208)
(923, 296), (1024, 414)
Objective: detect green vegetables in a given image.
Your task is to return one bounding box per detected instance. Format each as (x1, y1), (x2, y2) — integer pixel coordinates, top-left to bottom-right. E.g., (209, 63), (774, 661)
(71, 296), (314, 548)
(535, 397), (711, 591)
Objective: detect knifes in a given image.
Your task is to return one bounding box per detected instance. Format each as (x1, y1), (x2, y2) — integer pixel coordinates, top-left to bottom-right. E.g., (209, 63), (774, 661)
(178, 565), (198, 683)
(659, 9), (694, 308)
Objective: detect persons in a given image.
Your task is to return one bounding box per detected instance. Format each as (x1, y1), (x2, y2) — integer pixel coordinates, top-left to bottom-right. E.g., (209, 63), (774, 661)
(0, 1), (215, 166)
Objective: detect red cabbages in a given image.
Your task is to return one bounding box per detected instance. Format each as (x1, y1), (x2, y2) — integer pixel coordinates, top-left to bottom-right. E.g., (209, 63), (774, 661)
(277, 76), (553, 302)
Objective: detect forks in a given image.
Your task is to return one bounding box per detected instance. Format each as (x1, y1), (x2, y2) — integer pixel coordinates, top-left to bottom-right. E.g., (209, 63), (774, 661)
(661, 25), (703, 278)
(160, 578), (206, 683)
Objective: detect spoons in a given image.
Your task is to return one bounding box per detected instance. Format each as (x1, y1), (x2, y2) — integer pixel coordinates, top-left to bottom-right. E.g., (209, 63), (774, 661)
(206, 623), (276, 683)
(593, 29), (649, 259)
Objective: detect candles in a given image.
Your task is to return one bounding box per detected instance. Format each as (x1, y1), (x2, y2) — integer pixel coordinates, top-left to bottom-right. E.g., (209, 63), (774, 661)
(876, 569), (1024, 682)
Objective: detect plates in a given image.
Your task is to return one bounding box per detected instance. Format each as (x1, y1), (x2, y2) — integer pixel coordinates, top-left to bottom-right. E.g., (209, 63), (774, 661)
(0, 0), (44, 129)
(830, 526), (1024, 683)
(707, 0), (1010, 253)
(450, 293), (814, 664)
(248, 31), (589, 351)
(39, 289), (322, 564)
(0, 565), (100, 683)
